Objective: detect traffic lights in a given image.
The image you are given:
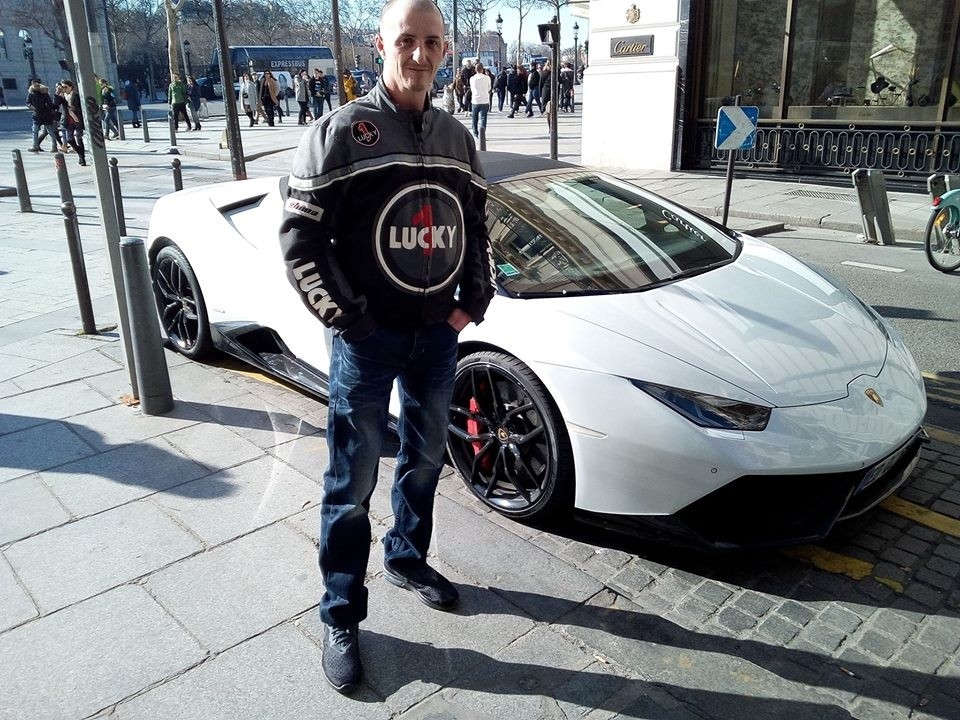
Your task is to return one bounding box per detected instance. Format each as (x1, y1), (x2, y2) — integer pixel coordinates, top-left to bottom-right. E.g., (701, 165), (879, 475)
(536, 24), (558, 46)
(375, 58), (384, 64)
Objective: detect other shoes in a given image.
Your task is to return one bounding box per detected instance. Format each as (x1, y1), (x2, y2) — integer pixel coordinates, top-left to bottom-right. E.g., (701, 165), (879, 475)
(508, 114), (515, 118)
(59, 144), (67, 152)
(61, 145), (70, 148)
(104, 132), (109, 139)
(28, 144), (39, 152)
(133, 124), (137, 128)
(37, 145), (44, 151)
(112, 131), (119, 138)
(74, 146), (86, 166)
(136, 122), (141, 126)
(528, 112), (534, 117)
(50, 144), (58, 152)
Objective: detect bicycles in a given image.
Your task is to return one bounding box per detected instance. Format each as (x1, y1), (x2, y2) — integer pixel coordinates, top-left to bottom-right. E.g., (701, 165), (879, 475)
(922, 188), (960, 274)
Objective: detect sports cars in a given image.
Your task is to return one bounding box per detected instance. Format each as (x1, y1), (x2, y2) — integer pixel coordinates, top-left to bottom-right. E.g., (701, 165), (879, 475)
(144, 148), (932, 557)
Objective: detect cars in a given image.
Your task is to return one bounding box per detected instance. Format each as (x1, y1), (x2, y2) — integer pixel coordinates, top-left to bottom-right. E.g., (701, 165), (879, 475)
(432, 66), (453, 90)
(333, 70), (377, 95)
(188, 77), (211, 98)
(521, 63), (530, 70)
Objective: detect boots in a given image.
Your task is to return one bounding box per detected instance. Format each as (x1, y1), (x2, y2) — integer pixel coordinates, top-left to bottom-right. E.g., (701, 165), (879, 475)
(246, 111), (255, 127)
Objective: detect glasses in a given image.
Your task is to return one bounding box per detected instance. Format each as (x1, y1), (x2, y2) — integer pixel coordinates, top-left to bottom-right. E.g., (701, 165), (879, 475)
(62, 86), (67, 88)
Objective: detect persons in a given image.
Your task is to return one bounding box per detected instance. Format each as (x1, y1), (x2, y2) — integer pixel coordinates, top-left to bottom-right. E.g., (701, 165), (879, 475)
(25, 73), (118, 153)
(0, 86), (6, 106)
(452, 58), (585, 118)
(930, 78), (957, 107)
(280, 0), (495, 695)
(343, 68), (372, 102)
(125, 78), (142, 127)
(52, 79), (87, 166)
(279, 74), (287, 94)
(294, 68), (332, 125)
(239, 68), (285, 127)
(168, 73), (209, 133)
(469, 64), (492, 139)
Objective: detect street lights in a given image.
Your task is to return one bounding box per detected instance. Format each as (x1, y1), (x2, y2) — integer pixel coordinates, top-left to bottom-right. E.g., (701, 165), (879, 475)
(573, 21), (580, 84)
(369, 42), (377, 70)
(24, 34), (38, 83)
(182, 39), (193, 74)
(496, 11), (504, 72)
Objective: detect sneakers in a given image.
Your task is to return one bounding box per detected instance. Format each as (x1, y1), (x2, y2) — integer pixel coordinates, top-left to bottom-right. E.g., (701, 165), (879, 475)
(382, 561), (458, 610)
(321, 623), (362, 690)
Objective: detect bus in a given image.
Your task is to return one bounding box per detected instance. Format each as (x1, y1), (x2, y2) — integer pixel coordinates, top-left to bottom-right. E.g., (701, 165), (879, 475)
(206, 45), (337, 97)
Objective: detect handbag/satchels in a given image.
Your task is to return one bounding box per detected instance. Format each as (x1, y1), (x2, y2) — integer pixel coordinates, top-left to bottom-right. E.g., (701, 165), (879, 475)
(351, 84), (361, 94)
(243, 93), (248, 98)
(54, 109), (62, 121)
